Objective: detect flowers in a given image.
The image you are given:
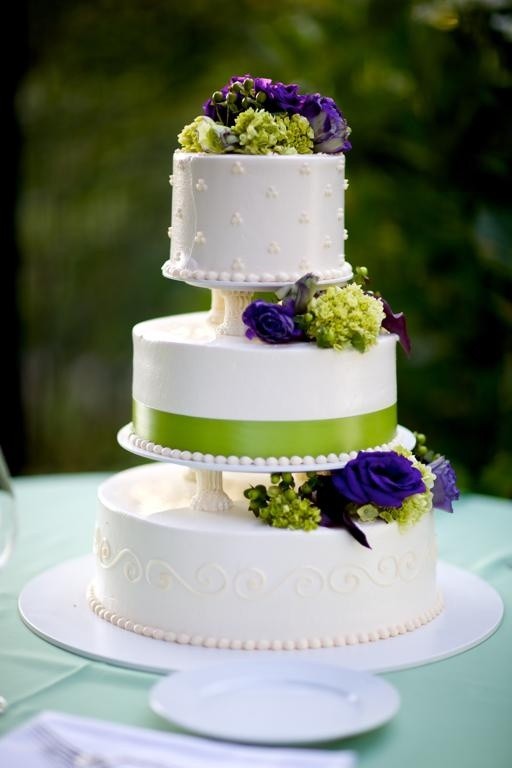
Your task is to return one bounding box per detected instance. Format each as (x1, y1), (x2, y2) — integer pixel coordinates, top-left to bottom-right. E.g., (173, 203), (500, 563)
(243, 431), (460, 551)
(177, 73), (352, 155)
(241, 265), (412, 355)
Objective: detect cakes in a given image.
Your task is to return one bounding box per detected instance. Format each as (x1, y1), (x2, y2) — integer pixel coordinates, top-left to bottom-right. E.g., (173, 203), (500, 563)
(88, 74), (459, 650)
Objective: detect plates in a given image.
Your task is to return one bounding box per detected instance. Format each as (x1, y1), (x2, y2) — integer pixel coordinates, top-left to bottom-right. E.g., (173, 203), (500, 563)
(147, 660), (400, 745)
